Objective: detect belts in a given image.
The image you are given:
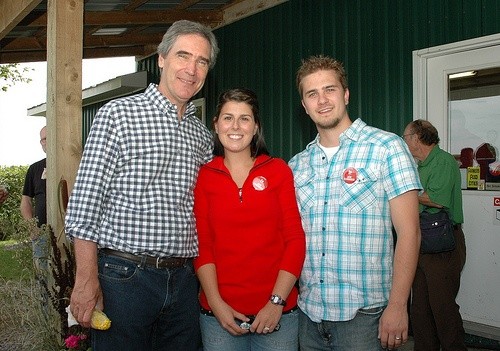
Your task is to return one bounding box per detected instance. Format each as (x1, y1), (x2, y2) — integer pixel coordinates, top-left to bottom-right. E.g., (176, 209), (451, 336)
(96, 248), (194, 268)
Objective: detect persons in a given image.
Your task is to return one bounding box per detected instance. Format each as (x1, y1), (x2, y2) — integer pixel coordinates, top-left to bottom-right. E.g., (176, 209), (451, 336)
(20, 125), (56, 322)
(401, 119), (469, 351)
(288, 55), (424, 351)
(193, 88), (306, 351)
(64, 20), (221, 351)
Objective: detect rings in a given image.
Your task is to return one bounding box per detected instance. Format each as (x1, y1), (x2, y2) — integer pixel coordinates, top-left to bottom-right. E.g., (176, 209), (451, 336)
(395, 337), (400, 340)
(264, 328), (269, 330)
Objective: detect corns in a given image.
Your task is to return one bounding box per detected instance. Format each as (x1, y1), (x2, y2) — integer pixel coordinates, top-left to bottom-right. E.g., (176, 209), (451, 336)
(90, 306), (112, 330)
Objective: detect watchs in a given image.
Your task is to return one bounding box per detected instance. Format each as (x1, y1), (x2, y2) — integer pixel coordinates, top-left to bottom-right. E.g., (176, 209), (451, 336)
(269, 294), (286, 306)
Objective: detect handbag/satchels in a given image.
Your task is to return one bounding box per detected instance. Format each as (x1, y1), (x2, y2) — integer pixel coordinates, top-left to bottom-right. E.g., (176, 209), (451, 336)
(419, 203), (456, 254)
